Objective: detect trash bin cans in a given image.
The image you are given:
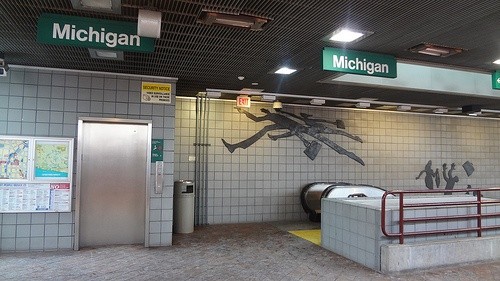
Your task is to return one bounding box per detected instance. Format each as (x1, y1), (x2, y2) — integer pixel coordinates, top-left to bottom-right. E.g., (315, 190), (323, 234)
(174, 179), (196, 235)
